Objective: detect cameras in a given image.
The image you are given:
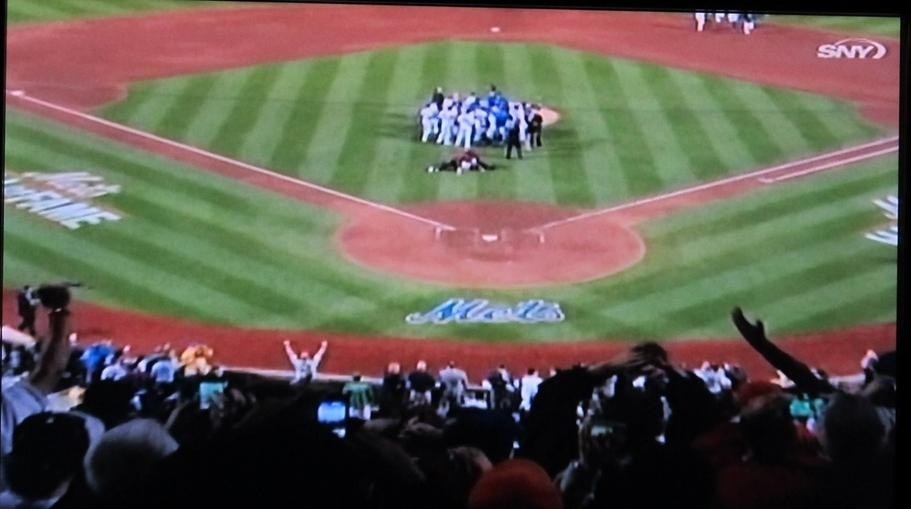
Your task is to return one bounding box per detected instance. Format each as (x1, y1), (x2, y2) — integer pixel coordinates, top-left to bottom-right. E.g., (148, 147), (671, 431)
(199, 382), (226, 412)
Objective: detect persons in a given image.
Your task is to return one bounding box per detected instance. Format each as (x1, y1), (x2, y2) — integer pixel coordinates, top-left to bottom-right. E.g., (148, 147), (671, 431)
(1, 277), (899, 509)
(695, 10), (766, 37)
(416, 84), (546, 176)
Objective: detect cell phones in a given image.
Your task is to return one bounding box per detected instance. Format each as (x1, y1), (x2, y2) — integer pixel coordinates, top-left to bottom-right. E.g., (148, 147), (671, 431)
(318, 395), (347, 441)
